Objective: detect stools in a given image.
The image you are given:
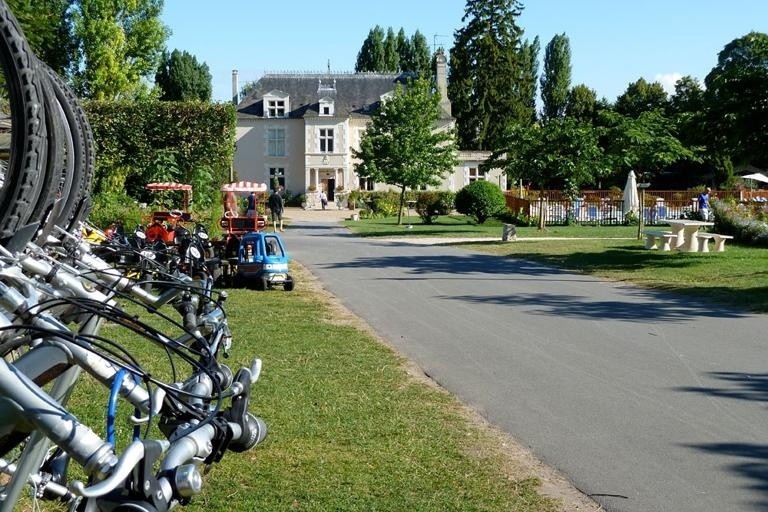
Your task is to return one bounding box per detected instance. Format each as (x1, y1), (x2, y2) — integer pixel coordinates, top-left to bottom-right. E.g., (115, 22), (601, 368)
(639, 230), (678, 252)
(695, 232), (735, 252)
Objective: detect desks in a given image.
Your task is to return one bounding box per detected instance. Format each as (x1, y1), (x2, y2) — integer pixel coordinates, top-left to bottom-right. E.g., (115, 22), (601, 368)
(657, 219), (716, 253)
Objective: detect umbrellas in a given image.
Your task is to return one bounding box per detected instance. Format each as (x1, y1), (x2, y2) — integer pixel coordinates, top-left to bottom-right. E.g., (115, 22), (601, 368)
(622, 169), (639, 216)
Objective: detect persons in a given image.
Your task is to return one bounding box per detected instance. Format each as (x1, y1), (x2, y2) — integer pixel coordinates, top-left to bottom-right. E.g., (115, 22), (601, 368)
(223, 191), (234, 217)
(319, 190), (328, 211)
(697, 187), (712, 230)
(246, 192), (259, 228)
(269, 188), (285, 234)
